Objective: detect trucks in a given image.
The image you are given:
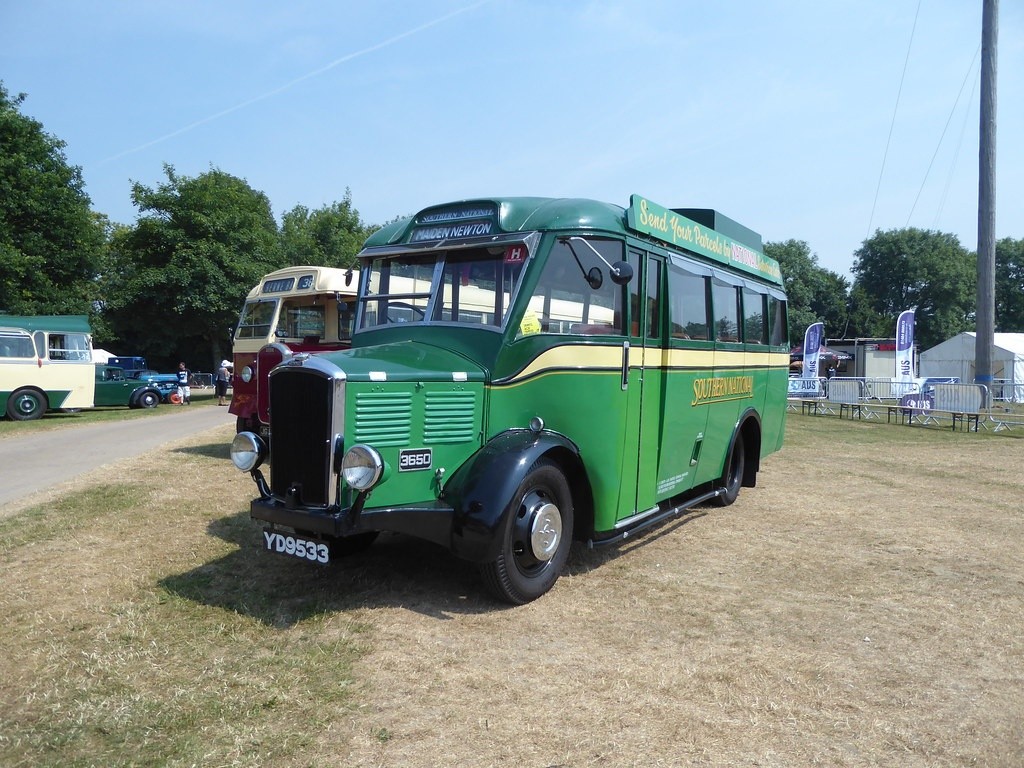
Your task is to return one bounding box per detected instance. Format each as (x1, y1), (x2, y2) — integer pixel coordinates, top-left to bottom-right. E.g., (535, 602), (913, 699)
(108, 356), (147, 370)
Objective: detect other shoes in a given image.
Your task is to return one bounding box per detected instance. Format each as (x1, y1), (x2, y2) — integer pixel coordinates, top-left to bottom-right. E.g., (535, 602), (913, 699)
(187, 403), (190, 405)
(177, 403), (183, 406)
(218, 403), (229, 406)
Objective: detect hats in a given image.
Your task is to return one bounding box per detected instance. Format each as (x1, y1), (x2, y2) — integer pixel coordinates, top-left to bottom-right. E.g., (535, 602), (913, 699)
(221, 359), (230, 367)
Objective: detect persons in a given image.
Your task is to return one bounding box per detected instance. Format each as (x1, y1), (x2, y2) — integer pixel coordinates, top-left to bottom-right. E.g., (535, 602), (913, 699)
(827, 364), (835, 378)
(216, 360), (231, 406)
(177, 363), (192, 406)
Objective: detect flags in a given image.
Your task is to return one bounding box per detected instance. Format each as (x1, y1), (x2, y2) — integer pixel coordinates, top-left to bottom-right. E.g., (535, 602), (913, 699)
(896, 311), (913, 382)
(803, 323), (823, 379)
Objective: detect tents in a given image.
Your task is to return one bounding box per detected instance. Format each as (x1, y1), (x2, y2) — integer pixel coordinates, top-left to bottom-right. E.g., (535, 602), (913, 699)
(93, 349), (118, 362)
(919, 333), (1024, 402)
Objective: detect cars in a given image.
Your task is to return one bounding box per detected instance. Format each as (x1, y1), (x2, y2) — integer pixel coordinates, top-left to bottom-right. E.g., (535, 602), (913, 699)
(124, 370), (181, 404)
(94, 366), (163, 409)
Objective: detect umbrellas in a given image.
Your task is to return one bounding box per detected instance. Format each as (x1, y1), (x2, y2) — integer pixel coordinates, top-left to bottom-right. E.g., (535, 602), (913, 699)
(790, 342), (852, 360)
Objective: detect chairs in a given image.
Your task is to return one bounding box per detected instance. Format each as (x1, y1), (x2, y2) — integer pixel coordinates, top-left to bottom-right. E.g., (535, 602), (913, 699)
(571, 322), (763, 344)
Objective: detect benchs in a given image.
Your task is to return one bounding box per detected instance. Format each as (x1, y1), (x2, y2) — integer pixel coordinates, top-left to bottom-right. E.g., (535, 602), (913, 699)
(800, 399), (1024, 434)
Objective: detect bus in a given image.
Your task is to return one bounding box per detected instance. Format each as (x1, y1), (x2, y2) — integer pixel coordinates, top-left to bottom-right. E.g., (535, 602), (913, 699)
(231, 196), (791, 607)
(228, 266), (618, 435)
(0, 315), (95, 420)
